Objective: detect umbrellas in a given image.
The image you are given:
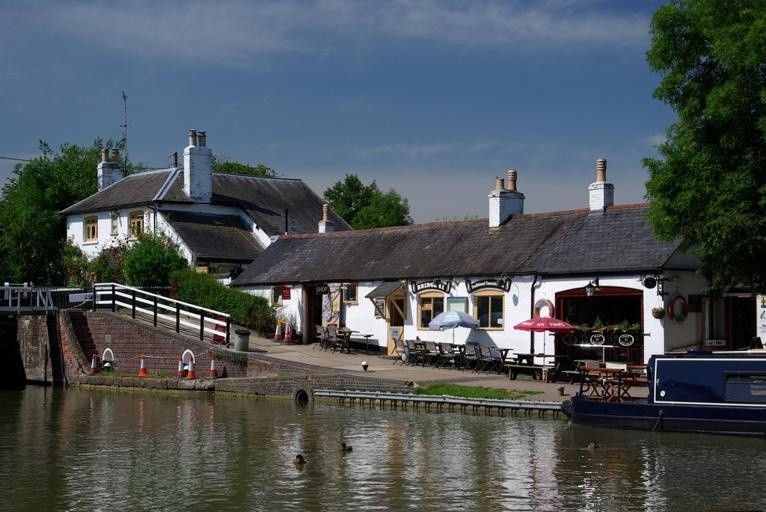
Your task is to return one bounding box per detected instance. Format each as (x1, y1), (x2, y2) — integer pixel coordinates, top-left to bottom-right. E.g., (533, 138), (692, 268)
(426, 310), (478, 356)
(514, 317), (576, 365)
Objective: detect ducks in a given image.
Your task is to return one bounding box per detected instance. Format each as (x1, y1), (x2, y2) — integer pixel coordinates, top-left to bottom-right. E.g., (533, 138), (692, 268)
(340, 441), (353, 451)
(291, 454), (307, 465)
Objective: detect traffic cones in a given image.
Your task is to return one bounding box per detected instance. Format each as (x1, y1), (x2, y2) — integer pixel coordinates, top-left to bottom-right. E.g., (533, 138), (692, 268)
(176, 354), (187, 377)
(184, 355), (198, 380)
(205, 353), (222, 379)
(136, 356), (149, 378)
(271, 319), (283, 342)
(90, 353), (99, 373)
(281, 323), (295, 344)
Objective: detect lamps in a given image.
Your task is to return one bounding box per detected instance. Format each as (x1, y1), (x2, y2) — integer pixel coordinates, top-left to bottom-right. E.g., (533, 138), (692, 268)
(644, 277), (669, 299)
(584, 278), (599, 296)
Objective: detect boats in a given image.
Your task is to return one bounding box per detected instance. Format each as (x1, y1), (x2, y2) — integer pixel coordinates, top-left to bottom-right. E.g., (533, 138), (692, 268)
(562, 350), (765, 436)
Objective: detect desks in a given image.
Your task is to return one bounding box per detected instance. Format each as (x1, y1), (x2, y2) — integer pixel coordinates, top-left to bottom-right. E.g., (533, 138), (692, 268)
(497, 348), (513, 375)
(513, 353), (568, 380)
(571, 360), (648, 403)
(317, 330), (360, 353)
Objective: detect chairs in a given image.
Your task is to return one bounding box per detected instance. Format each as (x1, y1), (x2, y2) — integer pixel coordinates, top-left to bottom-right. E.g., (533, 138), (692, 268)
(393, 337), (501, 375)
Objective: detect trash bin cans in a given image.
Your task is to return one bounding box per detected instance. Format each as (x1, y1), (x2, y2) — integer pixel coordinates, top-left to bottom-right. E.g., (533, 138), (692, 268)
(235, 329), (250, 352)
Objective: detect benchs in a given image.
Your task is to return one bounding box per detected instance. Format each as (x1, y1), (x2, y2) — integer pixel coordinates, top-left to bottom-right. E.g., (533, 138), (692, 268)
(338, 334), (373, 353)
(313, 336), (342, 353)
(505, 364), (612, 386)
